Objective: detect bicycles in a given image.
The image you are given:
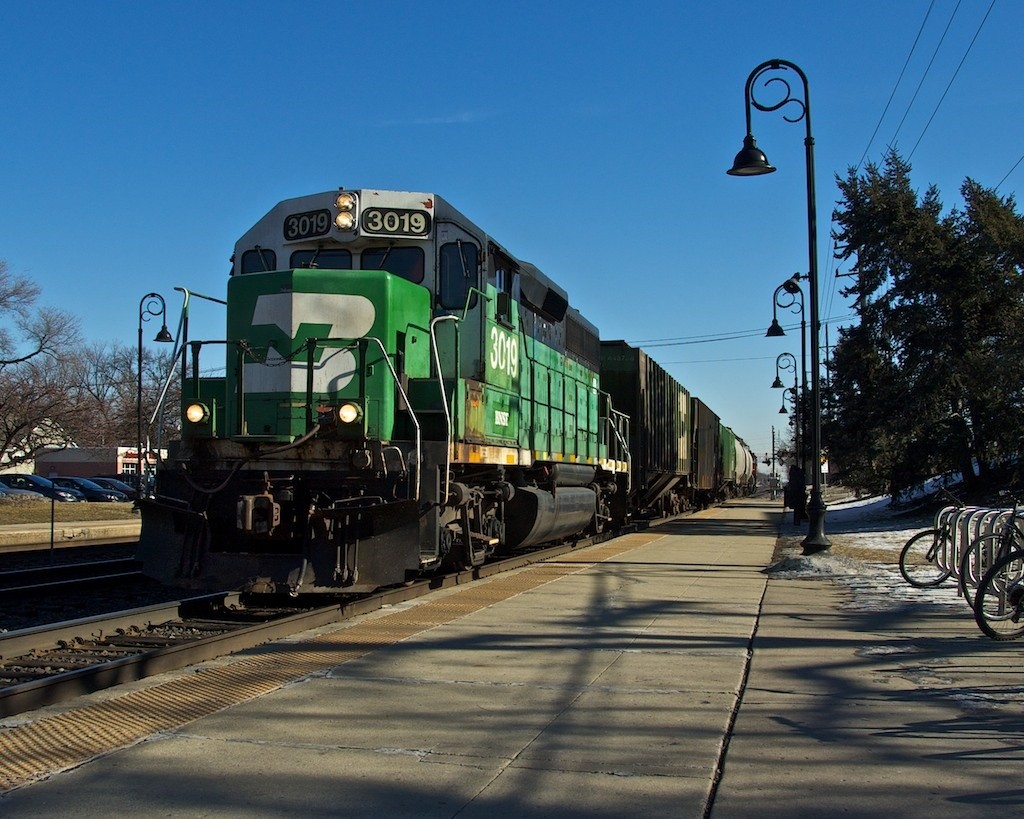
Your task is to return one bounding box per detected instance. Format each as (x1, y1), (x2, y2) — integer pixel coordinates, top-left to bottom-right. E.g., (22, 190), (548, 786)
(957, 490), (1024, 643)
(899, 487), (970, 588)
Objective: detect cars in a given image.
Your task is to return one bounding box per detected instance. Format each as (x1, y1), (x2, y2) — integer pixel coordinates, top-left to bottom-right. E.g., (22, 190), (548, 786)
(85, 477), (136, 501)
(43, 476), (129, 503)
(0, 473), (89, 504)
(0, 481), (45, 500)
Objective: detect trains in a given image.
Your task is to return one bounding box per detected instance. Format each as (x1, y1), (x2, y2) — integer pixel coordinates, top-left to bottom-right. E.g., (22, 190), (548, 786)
(131, 187), (758, 602)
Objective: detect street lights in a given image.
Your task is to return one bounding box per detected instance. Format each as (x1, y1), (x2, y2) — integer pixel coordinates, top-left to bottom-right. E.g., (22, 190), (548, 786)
(724, 59), (835, 558)
(135, 292), (177, 505)
(762, 281), (809, 526)
